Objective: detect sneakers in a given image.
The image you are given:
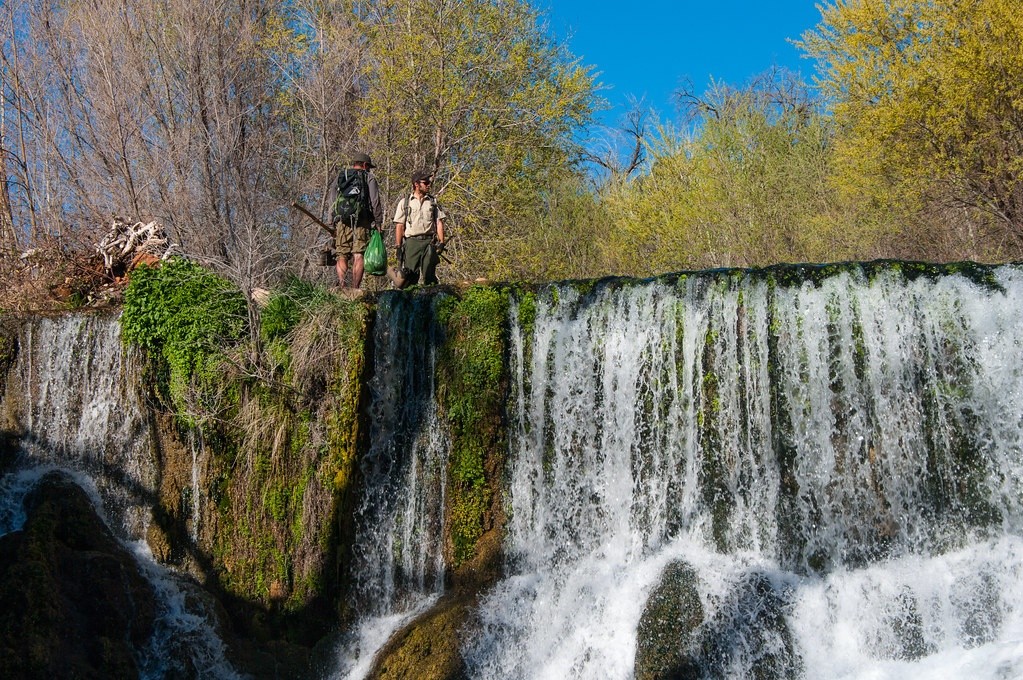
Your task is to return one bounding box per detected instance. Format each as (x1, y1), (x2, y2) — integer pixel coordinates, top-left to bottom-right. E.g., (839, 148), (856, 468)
(387, 267), (410, 289)
(318, 250), (337, 266)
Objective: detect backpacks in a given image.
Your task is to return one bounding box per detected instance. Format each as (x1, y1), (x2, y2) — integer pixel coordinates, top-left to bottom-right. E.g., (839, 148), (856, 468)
(334, 170), (370, 223)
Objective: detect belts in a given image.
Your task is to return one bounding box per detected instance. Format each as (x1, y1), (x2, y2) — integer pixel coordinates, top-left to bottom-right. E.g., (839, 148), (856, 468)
(410, 234), (433, 240)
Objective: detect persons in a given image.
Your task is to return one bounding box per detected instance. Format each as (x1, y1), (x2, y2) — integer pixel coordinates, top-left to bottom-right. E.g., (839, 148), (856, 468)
(393, 173), (447, 287)
(327, 153), (383, 290)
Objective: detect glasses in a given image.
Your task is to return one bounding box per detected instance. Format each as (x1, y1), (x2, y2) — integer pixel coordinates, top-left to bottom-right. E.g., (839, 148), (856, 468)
(420, 181), (430, 185)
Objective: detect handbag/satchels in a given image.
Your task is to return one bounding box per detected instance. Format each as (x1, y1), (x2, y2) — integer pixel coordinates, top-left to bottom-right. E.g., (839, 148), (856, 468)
(363, 230), (388, 275)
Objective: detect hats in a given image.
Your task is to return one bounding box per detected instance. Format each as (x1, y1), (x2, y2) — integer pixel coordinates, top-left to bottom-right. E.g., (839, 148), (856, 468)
(353, 153), (375, 168)
(412, 172), (433, 183)
